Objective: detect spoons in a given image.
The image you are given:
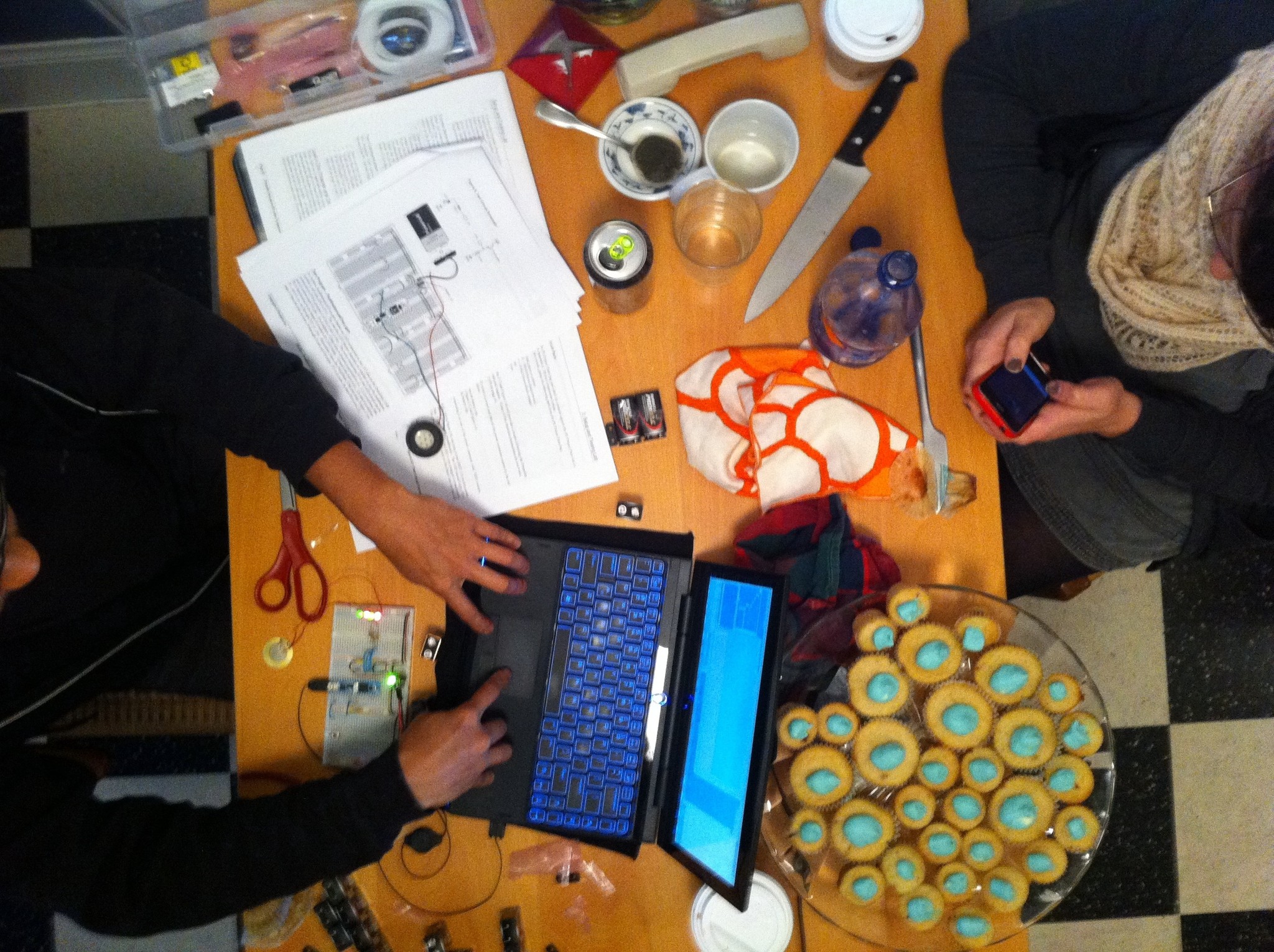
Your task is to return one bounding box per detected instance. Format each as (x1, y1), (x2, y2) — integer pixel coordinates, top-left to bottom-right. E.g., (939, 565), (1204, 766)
(534, 100), (683, 186)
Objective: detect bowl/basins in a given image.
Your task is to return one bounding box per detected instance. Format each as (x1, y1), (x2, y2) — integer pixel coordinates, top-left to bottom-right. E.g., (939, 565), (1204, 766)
(594, 96), (704, 202)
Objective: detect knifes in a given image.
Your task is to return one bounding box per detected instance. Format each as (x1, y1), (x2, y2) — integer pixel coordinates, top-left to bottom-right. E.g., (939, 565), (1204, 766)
(743, 58), (922, 327)
(905, 314), (949, 515)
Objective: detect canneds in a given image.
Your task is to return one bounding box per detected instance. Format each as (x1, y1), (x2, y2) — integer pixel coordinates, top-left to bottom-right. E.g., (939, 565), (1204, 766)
(582, 219), (654, 314)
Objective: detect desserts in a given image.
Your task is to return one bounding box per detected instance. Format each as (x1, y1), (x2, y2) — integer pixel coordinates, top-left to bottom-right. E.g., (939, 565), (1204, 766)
(776, 586), (1106, 946)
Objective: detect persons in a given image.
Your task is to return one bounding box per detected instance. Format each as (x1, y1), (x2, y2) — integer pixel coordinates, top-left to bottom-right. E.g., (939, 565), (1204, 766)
(947, 35), (1274, 602)
(0, 267), (531, 937)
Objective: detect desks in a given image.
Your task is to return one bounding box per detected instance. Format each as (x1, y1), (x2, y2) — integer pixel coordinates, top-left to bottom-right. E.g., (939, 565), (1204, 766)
(205, 0), (1028, 952)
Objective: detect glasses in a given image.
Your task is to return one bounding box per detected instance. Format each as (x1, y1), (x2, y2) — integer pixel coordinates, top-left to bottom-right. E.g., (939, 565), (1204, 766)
(1206, 162), (1274, 348)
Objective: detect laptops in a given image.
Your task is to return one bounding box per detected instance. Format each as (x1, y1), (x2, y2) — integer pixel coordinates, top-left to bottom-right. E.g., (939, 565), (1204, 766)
(439, 536), (787, 912)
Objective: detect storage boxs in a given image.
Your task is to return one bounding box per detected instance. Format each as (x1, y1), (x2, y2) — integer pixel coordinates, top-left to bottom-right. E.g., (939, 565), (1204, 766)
(122, 0), (495, 157)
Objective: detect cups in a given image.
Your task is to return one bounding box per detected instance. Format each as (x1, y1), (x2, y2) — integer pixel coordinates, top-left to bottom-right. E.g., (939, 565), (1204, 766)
(672, 182), (762, 287)
(688, 869), (795, 952)
(702, 98), (799, 202)
(818, 0), (928, 93)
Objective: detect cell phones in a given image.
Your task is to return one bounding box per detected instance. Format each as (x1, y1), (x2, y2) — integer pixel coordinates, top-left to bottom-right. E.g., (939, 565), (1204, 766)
(971, 351), (1055, 441)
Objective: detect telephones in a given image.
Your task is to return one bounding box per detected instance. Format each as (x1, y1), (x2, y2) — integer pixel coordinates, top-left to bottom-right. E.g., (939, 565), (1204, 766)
(615, 4), (808, 101)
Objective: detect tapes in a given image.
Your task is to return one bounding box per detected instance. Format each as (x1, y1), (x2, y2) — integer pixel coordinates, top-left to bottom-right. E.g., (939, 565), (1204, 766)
(358, 0), (455, 74)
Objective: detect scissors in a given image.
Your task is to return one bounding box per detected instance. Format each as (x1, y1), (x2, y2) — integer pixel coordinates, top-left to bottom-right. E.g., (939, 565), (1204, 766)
(254, 471), (328, 621)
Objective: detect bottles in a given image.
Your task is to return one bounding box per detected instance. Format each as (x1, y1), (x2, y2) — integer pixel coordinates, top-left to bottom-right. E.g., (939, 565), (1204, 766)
(810, 248), (926, 370)
